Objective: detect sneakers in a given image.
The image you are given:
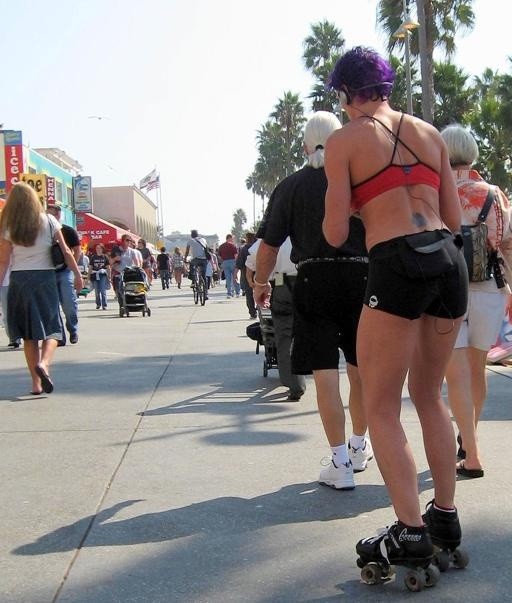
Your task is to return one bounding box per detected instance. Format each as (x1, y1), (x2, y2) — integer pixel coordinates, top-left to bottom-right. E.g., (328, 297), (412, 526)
(96, 305), (100, 310)
(8, 343), (19, 349)
(288, 391), (304, 400)
(70, 333), (78, 344)
(319, 456), (355, 490)
(347, 438), (373, 473)
(103, 307), (108, 310)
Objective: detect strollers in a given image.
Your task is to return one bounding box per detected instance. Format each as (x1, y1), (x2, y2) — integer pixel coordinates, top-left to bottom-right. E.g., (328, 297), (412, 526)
(118, 262), (151, 317)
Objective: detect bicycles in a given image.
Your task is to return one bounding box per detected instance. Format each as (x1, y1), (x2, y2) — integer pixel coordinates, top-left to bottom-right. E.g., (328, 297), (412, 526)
(185, 260), (206, 305)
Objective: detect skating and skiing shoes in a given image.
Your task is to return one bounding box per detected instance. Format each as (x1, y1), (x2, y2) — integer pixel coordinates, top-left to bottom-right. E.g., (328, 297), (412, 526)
(421, 498), (469, 572)
(356, 520), (440, 592)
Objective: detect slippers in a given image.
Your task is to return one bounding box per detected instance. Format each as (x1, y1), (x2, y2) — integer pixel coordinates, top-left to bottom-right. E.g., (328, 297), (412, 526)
(30, 390), (44, 395)
(456, 459), (484, 478)
(35, 366), (53, 393)
(457, 432), (466, 459)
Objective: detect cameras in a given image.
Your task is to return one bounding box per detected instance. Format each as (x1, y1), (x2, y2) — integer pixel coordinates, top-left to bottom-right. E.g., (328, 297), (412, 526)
(492, 263), (506, 289)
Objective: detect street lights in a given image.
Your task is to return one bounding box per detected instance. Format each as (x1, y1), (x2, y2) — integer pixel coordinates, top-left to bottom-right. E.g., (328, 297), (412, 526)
(392, 1), (421, 117)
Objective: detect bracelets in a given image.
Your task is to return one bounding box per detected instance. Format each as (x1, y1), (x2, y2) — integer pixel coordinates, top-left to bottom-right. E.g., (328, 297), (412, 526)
(250, 272), (272, 287)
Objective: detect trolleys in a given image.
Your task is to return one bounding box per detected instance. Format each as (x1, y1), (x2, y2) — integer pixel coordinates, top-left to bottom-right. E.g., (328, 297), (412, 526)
(211, 261), (221, 285)
(253, 297), (279, 378)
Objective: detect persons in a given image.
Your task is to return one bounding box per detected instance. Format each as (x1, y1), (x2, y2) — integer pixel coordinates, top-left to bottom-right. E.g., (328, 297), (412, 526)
(235, 224), (307, 403)
(249, 106), (373, 491)
(439, 123), (511, 472)
(320, 43), (469, 568)
(1, 179), (83, 395)
(184, 231), (258, 299)
(78, 234), (186, 309)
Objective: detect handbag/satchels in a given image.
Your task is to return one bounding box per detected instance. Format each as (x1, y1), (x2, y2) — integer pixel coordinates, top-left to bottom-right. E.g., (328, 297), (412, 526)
(205, 245), (214, 260)
(460, 222), (489, 282)
(51, 244), (63, 266)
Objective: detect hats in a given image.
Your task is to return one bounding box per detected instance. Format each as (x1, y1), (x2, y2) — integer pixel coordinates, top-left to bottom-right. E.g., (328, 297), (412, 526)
(47, 204), (60, 210)
